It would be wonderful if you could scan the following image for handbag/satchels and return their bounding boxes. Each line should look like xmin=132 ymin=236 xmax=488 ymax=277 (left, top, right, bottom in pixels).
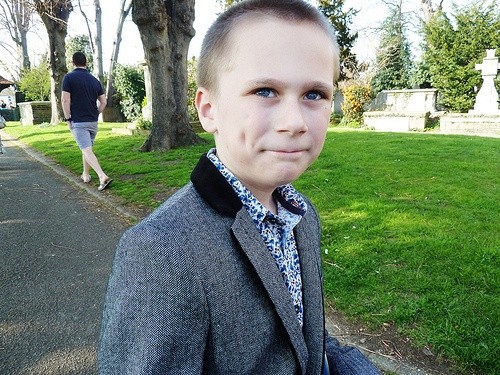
xmin=0 ymin=116 xmax=6 ymax=128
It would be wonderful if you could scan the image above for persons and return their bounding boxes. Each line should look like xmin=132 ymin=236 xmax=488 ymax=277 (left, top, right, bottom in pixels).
xmin=1 ymin=102 xmax=6 ymax=108
xmin=61 ymin=52 xmax=114 ymax=191
xmin=0 ymin=108 xmax=6 ymax=154
xmin=97 ymin=0 xmax=389 ymax=375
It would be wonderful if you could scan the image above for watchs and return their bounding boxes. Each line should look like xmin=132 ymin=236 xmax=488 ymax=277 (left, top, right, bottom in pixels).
xmin=64 ymin=118 xmax=71 ymax=122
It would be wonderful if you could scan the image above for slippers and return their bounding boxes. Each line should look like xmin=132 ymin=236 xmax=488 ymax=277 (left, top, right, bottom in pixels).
xmin=98 ymin=178 xmax=113 ymax=191
xmin=81 ymin=174 xmax=91 ymax=183
